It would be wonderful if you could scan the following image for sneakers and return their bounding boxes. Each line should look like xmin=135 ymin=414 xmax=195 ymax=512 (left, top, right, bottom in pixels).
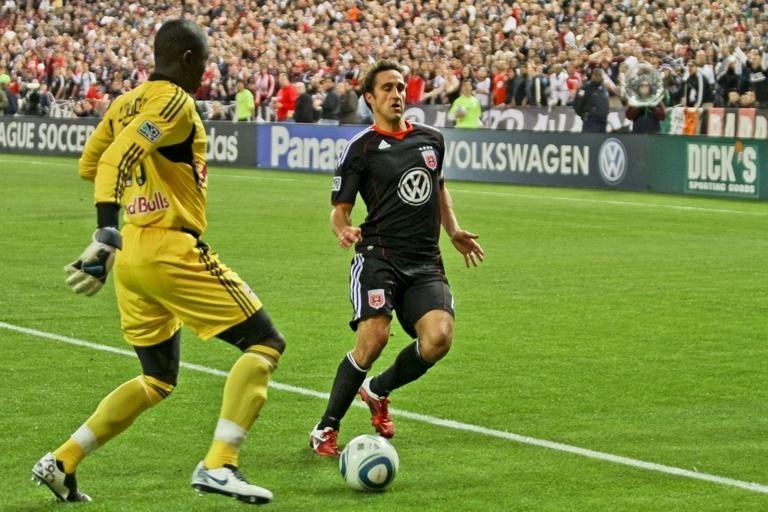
xmin=360 ymin=376 xmax=396 ymax=438
xmin=309 ymin=421 xmax=342 ymax=459
xmin=31 ymin=452 xmax=93 ymax=504
xmin=189 ymin=460 xmax=273 ymax=506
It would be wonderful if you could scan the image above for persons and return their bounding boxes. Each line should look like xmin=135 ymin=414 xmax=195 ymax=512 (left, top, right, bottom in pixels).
xmin=1 ymin=0 xmax=768 ymax=133
xmin=307 ymin=59 xmax=485 ymax=456
xmin=31 ymin=16 xmax=287 ymax=505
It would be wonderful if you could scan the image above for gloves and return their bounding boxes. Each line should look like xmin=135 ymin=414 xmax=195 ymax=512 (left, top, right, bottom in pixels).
xmin=63 ymin=227 xmax=124 ymax=298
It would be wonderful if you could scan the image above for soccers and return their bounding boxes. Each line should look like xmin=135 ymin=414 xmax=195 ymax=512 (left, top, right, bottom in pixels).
xmin=338 ymin=434 xmax=399 ymax=493
